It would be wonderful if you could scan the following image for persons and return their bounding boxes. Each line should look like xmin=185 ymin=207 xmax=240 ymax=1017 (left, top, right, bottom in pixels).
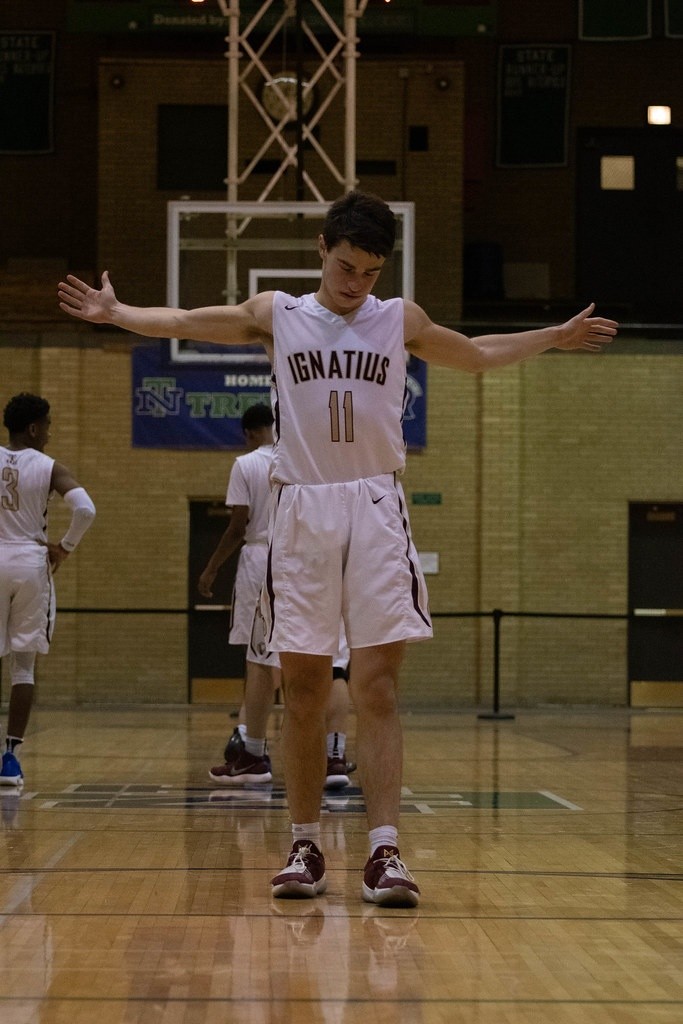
xmin=57 ymin=190 xmax=619 ymax=908
xmin=196 ymin=403 xmax=355 ymax=788
xmin=0 ymin=393 xmax=95 ymax=786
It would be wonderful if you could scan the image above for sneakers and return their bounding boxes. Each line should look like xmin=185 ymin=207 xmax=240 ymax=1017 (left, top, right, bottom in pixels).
xmin=361 ymin=845 xmax=421 ymax=905
xmin=324 ymin=755 xmax=349 ymax=788
xmin=361 ymin=901 xmax=420 ymax=962
xmin=270 ymin=840 xmax=327 ymax=897
xmin=209 ymin=789 xmax=270 ymax=826
xmin=320 ymin=795 xmax=349 ymax=814
xmin=207 ymin=748 xmax=273 ymax=783
xmin=1 ymin=786 xmax=23 ymax=820
xmin=345 ymin=761 xmax=357 ymax=774
xmin=269 ymin=905 xmax=325 ymax=948
xmin=0 ymin=753 xmax=24 ymax=786
xmin=225 ymin=727 xmax=271 ymax=770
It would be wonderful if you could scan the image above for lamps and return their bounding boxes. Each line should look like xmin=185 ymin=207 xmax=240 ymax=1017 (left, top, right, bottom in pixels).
xmin=257 ymin=0 xmax=319 ymax=125
